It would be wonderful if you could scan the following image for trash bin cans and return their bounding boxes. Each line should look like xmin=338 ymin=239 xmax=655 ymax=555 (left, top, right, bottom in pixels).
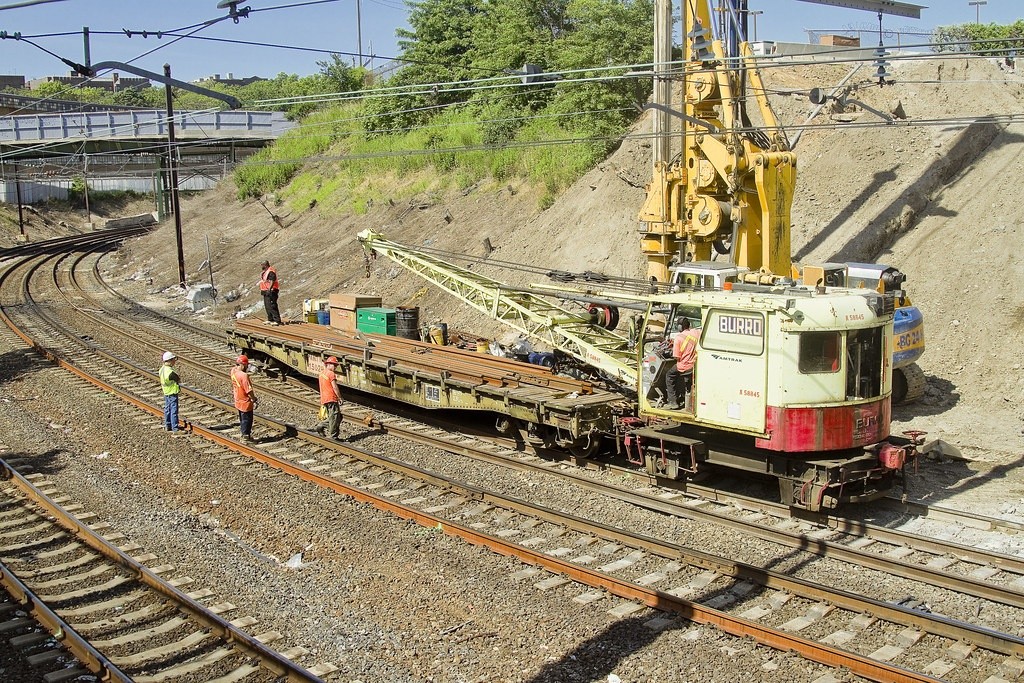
xmin=356 ymin=307 xmax=397 ymax=336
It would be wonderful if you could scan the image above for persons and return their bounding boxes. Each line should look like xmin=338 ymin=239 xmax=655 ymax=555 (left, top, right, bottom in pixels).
xmin=313 ymin=355 xmax=344 ymax=443
xmin=230 ymin=355 xmax=258 ymax=445
xmin=158 ymin=351 xmax=188 ymax=435
xmin=662 ymin=317 xmax=701 ymax=409
xmin=259 ymin=260 xmax=283 ymax=326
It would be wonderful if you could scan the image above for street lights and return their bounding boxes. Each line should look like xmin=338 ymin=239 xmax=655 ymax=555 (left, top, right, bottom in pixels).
xmin=967 ymin=0 xmax=988 ymax=24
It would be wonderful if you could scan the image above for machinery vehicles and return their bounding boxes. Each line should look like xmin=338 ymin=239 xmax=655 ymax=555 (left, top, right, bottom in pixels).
xmin=634 ymin=0 xmax=931 ymax=407
xmin=354 ymin=223 xmax=930 ymax=516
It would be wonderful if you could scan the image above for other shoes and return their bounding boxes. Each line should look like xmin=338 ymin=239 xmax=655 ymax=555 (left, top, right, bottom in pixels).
xmin=677 ymin=400 xmax=684 ymax=406
xmin=263 ymin=321 xmax=271 ymax=324
xmin=331 ymin=437 xmax=344 ymax=442
xmin=663 ymin=401 xmax=679 ymax=409
xmin=173 ymin=430 xmax=187 ymax=435
xmin=317 ymin=426 xmax=325 ymax=437
xmin=270 ymin=322 xmax=278 ymax=326
xmin=165 ymin=427 xmax=172 ymax=431
xmin=240 ymin=435 xmax=258 ymax=445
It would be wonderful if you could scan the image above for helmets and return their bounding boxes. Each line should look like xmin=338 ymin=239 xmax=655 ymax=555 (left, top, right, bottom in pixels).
xmin=324 ymin=356 xmax=340 ymax=365
xmin=163 ymin=351 xmax=176 ymax=362
xmin=236 ymin=355 xmax=249 ymax=365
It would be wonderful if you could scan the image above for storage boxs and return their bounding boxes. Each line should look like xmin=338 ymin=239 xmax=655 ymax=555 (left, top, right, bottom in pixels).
xmin=329 ymin=294 xmax=397 ymax=336
xmin=303 ymin=299 xmax=329 ymax=323
